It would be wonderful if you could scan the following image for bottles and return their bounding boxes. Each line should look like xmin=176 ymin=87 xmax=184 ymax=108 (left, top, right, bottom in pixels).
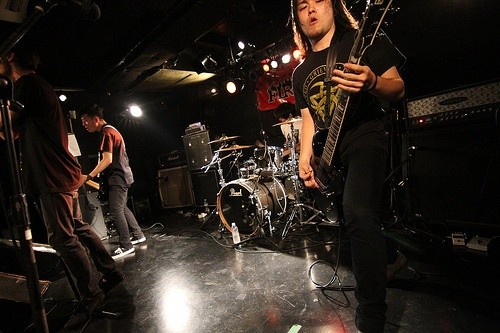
xmin=231 ymin=222 xmax=241 ymax=244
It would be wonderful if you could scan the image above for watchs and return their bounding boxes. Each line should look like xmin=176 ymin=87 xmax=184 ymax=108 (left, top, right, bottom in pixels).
xmin=87 ymin=174 xmax=93 ymax=180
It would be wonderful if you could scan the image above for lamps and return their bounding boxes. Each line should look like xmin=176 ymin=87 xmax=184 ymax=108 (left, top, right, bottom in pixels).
xmin=224 ymin=77 xmax=246 ymax=95
xmin=191 ymin=52 xmax=216 ymax=75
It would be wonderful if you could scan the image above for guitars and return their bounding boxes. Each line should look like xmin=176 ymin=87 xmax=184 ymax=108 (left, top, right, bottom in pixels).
xmin=305 ymin=1 xmax=393 ymax=223
xmin=85 ymin=178 xmax=102 ymax=194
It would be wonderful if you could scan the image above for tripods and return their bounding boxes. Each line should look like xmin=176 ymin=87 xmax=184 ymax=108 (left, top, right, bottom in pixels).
xmin=230 ymin=172 xmax=283 ymax=251
xmin=278 ymin=124 xmax=325 ymax=240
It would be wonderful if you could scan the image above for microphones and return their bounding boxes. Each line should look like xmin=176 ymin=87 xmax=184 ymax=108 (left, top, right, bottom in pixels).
xmin=73 ymin=1 xmax=101 ymax=21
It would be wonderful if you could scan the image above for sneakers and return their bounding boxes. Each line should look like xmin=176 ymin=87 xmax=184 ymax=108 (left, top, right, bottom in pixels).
xmin=96 ymin=269 xmax=126 ymax=293
xmin=64 ymin=291 xmax=106 ymax=329
xmin=378 ymin=250 xmax=408 ymax=283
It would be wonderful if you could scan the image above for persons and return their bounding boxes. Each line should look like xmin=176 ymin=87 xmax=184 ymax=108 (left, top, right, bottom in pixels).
xmin=74 ymin=106 xmax=146 ymax=260
xmin=274 ymin=101 xmax=301 ymax=140
xmin=286 ymin=0 xmax=403 ymax=333
xmin=7 ymin=42 xmax=126 ymax=309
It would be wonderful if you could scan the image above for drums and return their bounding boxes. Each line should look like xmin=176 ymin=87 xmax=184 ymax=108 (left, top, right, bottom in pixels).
xmin=215 ymin=171 xmax=286 ymax=239
xmin=282 ymin=173 xmax=304 ymax=201
xmin=256 ymin=146 xmax=286 ymax=178
xmin=239 ymin=174 xmax=258 ymax=179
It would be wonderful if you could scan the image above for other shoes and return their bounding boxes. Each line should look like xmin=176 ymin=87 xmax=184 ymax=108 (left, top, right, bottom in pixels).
xmin=129 ymin=234 xmax=147 ymax=245
xmin=110 ymin=246 xmax=135 ymax=261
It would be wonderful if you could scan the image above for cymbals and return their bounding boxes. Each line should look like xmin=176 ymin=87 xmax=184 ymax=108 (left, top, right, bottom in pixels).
xmin=214 ymin=144 xmax=253 ymax=154
xmin=207 ymin=135 xmax=240 ymax=145
xmin=272 ymin=119 xmax=302 ymax=126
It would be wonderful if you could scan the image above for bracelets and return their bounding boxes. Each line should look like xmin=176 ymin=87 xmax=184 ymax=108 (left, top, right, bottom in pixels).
xmin=369 ymin=76 xmax=377 ymax=92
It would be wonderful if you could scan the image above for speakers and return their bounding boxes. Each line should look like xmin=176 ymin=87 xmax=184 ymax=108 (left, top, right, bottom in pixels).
xmin=158 ymin=165 xmax=195 ymax=208
xmin=402 ymin=110 xmax=500 ymax=237
xmin=185 ymin=131 xmax=218 ymax=171
xmin=79 ymin=191 xmax=106 ymax=241
xmin=188 ymin=169 xmax=219 ymax=208
